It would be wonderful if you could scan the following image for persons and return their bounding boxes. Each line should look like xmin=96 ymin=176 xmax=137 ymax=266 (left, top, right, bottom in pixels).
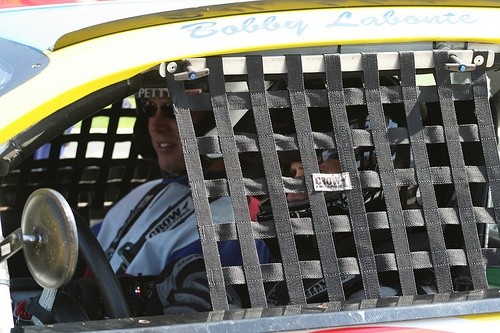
xmin=258 ymin=72 xmax=429 ymax=305
xmin=77 ymin=63 xmax=268 ymax=316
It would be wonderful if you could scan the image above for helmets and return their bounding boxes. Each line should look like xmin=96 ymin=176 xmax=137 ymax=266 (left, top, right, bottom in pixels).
xmin=232 ymin=75 xmax=425 ymax=256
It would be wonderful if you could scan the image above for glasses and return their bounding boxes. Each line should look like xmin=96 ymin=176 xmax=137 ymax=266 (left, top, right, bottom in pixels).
xmin=140 ymin=102 xmax=176 ymax=118
